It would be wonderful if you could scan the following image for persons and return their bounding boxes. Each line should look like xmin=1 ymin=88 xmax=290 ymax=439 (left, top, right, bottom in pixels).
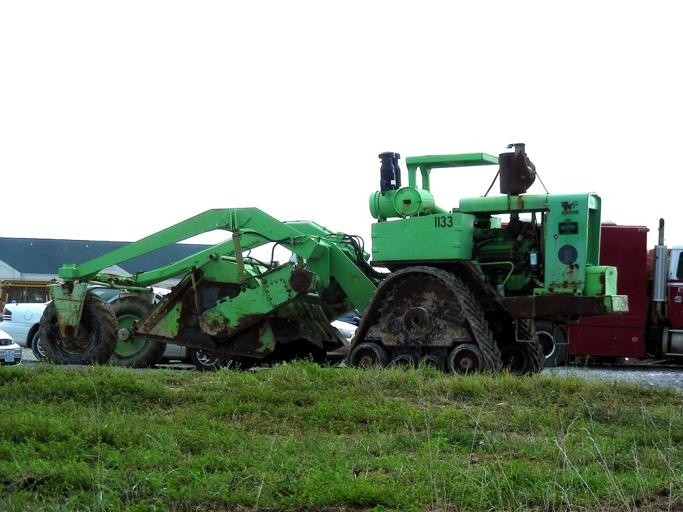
xmin=321 ymin=276 xmax=348 ymax=317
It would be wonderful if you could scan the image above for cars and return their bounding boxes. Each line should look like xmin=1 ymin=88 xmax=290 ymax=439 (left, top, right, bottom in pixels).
xmin=1 ymin=287 xmax=356 ymax=366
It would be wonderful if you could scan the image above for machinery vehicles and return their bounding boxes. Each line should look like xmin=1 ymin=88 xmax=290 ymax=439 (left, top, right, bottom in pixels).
xmin=41 ymin=144 xmax=628 ymax=375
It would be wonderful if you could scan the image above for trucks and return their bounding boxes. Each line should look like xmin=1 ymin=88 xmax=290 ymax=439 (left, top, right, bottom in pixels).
xmin=502 ymin=219 xmax=682 ymax=367
